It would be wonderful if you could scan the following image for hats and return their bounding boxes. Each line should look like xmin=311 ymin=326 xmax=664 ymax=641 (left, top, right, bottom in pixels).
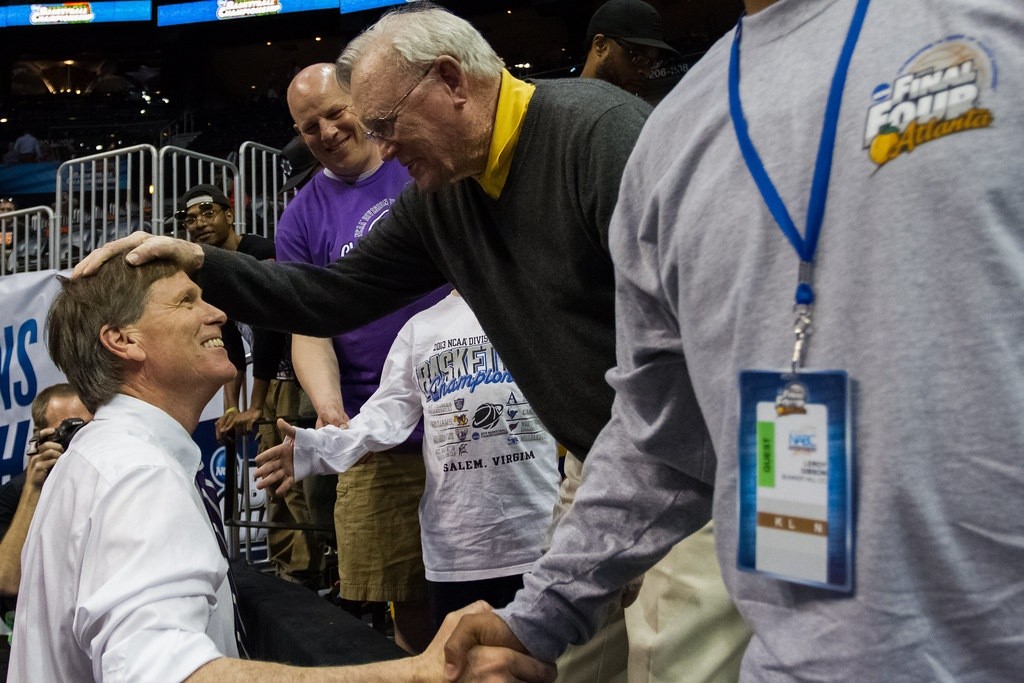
xmin=276 ymin=135 xmax=319 ymax=196
xmin=179 ymin=184 xmax=230 ymax=215
xmin=587 ymin=0 xmax=682 ymax=57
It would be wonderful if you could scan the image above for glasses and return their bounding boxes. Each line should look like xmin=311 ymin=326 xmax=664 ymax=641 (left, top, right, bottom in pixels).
xmin=605 ymin=37 xmax=652 ymax=68
xmin=175 ymin=203 xmax=226 ymax=228
xmin=360 ymin=53 xmax=450 ymax=145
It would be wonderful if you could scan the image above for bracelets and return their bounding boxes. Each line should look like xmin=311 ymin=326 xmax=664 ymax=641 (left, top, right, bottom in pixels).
xmin=224 ymin=407 xmax=239 ymax=415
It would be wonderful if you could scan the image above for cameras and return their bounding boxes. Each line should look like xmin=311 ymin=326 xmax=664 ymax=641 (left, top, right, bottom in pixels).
xmin=40 ymin=417 xmax=92 ymax=454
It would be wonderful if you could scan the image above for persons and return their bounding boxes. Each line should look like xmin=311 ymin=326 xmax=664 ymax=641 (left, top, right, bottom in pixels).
xmin=443 ymin=0 xmax=1024 ymax=683
xmin=0 ymin=0 xmax=657 ymax=683
xmin=4 ymin=246 xmax=560 ymax=683
xmin=13 ymin=127 xmax=42 ymax=162
